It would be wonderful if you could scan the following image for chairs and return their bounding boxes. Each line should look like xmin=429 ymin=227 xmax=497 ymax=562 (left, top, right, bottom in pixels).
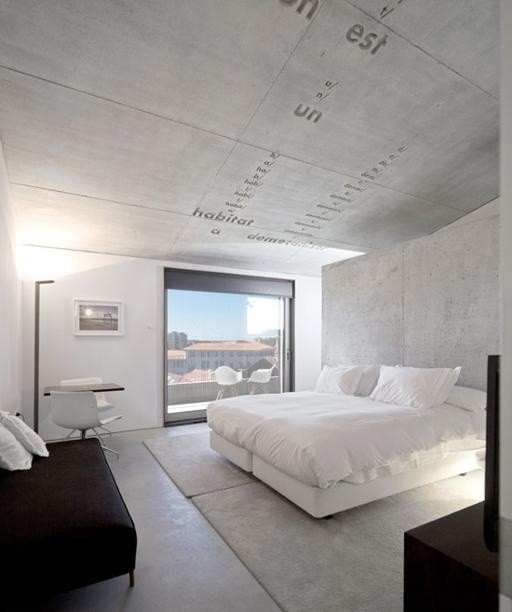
xmin=213 ymin=363 xmax=276 ymax=399
xmin=50 ymin=375 xmax=125 ymax=461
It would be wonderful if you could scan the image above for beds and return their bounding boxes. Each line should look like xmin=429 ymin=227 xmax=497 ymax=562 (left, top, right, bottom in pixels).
xmin=206 ymin=363 xmax=486 ymax=518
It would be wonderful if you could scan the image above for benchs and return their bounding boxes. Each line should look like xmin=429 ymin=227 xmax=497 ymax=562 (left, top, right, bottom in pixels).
xmin=1 ymin=437 xmax=137 ymax=609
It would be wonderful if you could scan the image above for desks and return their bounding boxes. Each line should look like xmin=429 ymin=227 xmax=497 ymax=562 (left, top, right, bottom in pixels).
xmin=43 ymin=381 xmax=125 ymax=396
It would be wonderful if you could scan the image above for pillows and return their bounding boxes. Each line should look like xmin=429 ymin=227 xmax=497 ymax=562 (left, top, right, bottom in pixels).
xmin=315 ymin=363 xmax=463 ymax=409
xmin=1 ymin=408 xmax=51 ymax=472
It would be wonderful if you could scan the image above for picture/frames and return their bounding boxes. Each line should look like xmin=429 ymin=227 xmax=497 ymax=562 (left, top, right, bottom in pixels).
xmin=72 ymin=297 xmax=126 ymax=336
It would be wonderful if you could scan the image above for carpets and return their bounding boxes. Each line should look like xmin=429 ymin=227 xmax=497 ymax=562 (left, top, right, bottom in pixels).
xmin=190 ymin=480 xmax=482 ymax=612
xmin=141 ymin=429 xmax=260 ymax=500
